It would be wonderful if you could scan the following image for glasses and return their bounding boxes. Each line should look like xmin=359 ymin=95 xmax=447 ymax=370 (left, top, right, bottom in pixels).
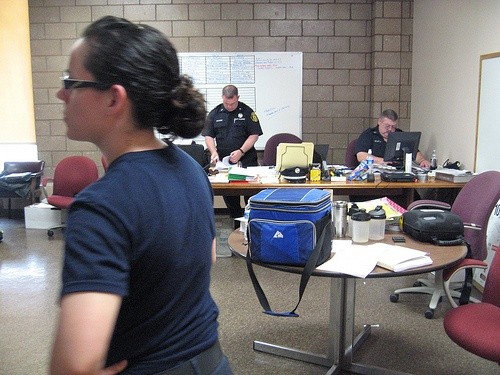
xmin=60 ymin=70 xmax=130 ymax=97
xmin=382 ymin=122 xmax=396 ymax=130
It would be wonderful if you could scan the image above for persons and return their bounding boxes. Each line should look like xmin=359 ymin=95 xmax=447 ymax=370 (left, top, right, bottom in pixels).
xmin=51 ymin=15 xmax=236 ymax=375
xmin=202 ymin=85 xmax=263 ymax=230
xmin=352 ymin=109 xmax=422 ymax=210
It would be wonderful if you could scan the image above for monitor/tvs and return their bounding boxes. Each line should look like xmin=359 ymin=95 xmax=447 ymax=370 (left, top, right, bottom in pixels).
xmin=385 ymin=131 xmax=421 ymax=173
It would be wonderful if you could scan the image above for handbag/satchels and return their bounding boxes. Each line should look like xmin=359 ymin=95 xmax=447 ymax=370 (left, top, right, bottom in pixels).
xmin=400 ymin=209 xmax=464 ymax=245
xmin=247 ymin=188 xmax=333 ymax=318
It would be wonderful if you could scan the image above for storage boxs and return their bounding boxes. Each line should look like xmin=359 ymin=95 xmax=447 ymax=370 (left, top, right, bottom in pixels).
xmin=24 ymin=203 xmax=63 ymax=229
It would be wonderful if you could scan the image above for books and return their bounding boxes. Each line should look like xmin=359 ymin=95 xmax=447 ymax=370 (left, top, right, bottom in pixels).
xmin=214 ymin=156 xmax=232 ymax=173
xmin=316 ymin=239 xmax=381 ymax=279
xmin=367 ymin=243 xmax=433 ymax=273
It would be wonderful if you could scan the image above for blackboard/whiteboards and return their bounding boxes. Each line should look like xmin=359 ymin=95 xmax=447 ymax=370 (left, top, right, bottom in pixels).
xmin=153 ymin=52 xmax=303 ymax=150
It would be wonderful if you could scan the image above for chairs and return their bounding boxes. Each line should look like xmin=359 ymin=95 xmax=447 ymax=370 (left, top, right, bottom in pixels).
xmin=442 ymin=241 xmax=500 ymax=366
xmin=263 ymin=133 xmax=361 ymax=168
xmin=0 ymin=159 xmax=45 ymax=217
xmin=42 ymin=156 xmax=98 ymax=237
xmin=389 ymin=170 xmax=500 ymax=319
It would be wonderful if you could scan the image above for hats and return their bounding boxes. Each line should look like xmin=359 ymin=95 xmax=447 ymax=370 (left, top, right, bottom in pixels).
xmin=281 ymin=166 xmax=308 ymax=183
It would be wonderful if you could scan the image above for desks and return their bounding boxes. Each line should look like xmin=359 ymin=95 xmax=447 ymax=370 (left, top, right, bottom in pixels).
xmin=227 ymin=221 xmax=469 ymax=375
xmin=207 ymin=167 xmax=472 ymax=210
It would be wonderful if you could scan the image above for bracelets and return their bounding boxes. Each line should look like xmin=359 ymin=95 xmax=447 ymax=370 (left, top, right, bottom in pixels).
xmin=239 ymin=148 xmax=245 ymax=156
xmin=419 ymin=159 xmax=427 ymax=165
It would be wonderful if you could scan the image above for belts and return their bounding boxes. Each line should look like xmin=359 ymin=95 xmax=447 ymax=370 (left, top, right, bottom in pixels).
xmin=154 ymin=341 xmax=224 ymax=375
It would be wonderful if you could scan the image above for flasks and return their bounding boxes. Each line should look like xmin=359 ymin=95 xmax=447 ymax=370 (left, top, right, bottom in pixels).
xmin=333 ymin=200 xmax=348 ymax=238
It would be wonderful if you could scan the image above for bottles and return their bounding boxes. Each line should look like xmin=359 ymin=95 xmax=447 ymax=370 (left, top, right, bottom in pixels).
xmin=368 ymin=205 xmax=386 ymax=241
xmin=430 ymin=150 xmax=437 ymax=170
xmin=365 ymin=149 xmax=374 ymax=165
xmin=348 ymin=204 xmax=359 ymax=238
xmin=351 ymin=208 xmax=372 ymax=243
